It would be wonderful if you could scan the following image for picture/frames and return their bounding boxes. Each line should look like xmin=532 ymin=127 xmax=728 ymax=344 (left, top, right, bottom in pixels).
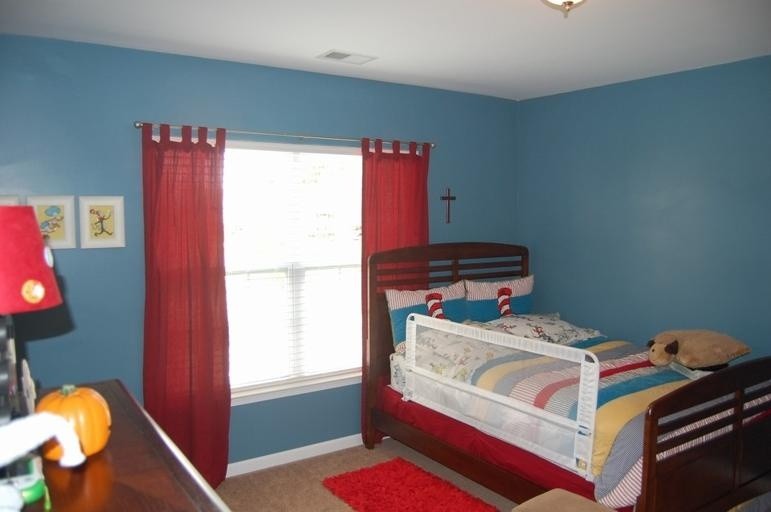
xmin=0 ymin=195 xmax=20 ymax=206
xmin=79 ymin=195 xmax=126 ymax=249
xmin=27 ymin=195 xmax=76 ymax=249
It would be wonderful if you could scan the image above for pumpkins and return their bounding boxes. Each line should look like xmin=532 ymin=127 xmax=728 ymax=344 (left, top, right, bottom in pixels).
xmin=40 ymin=450 xmax=117 ymax=511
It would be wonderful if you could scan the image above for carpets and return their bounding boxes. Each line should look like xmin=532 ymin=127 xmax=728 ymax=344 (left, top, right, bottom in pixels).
xmin=322 ymin=457 xmax=503 ymax=512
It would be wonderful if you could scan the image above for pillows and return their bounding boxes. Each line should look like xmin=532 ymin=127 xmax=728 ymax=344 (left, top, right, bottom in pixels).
xmin=395 ymin=319 xmax=522 ymax=377
xmin=481 ymin=312 xmax=604 ymax=348
xmin=653 ymin=327 xmax=751 ymax=368
xmin=463 ymin=274 xmax=535 ymax=325
xmin=384 ymin=280 xmax=466 ymax=346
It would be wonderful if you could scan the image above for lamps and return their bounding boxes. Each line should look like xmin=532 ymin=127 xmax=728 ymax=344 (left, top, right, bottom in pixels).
xmin=0 ymin=205 xmax=64 ymax=422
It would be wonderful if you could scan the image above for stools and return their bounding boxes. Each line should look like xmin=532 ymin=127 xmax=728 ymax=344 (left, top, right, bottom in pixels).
xmin=511 ymin=488 xmax=617 ymax=512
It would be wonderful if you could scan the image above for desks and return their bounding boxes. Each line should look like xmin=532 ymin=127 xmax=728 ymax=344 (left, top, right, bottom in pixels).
xmin=0 ymin=375 xmax=231 ymax=512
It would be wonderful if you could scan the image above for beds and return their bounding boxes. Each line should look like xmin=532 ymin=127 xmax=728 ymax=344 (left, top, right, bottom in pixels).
xmin=361 ymin=243 xmax=771 ymax=512
